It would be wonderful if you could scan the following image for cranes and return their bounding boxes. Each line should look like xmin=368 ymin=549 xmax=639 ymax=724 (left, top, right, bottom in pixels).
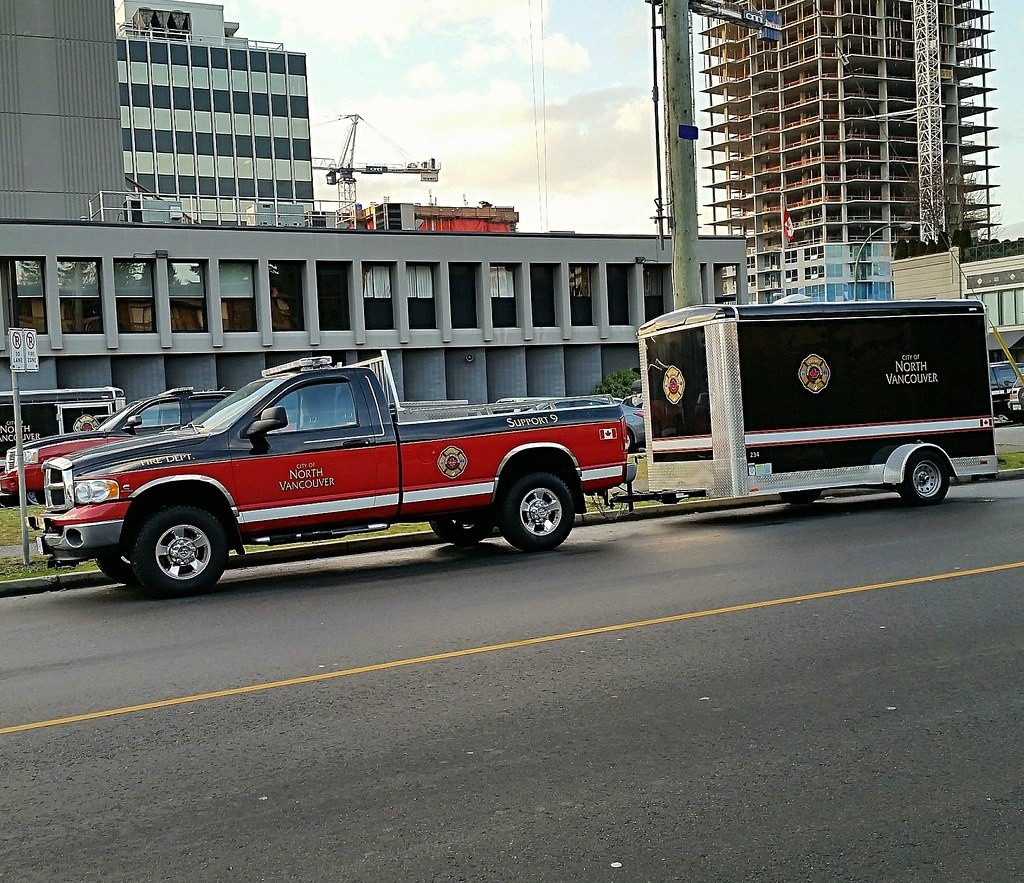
xmin=310 ymin=114 xmax=441 ymax=229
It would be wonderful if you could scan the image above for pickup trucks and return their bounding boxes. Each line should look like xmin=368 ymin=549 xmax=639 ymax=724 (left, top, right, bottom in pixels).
xmin=29 ymin=342 xmax=638 ymax=597
xmin=0 ymin=382 xmax=470 ymax=505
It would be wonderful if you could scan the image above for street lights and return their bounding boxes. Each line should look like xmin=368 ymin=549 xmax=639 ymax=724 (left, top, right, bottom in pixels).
xmin=854 ymin=221 xmax=912 ymax=303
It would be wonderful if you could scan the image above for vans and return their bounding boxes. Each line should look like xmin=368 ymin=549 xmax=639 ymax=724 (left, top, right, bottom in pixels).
xmin=491 ymin=396 xmax=624 ymax=413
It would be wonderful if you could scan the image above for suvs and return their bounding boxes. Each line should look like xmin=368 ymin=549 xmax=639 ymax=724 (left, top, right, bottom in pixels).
xmin=989 ymin=362 xmax=1024 ymax=416
xmin=1007 ymin=374 xmax=1023 ymax=425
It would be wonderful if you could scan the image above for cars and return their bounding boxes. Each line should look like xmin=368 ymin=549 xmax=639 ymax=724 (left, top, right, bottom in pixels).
xmin=520 ymin=397 xmax=644 ymax=453
